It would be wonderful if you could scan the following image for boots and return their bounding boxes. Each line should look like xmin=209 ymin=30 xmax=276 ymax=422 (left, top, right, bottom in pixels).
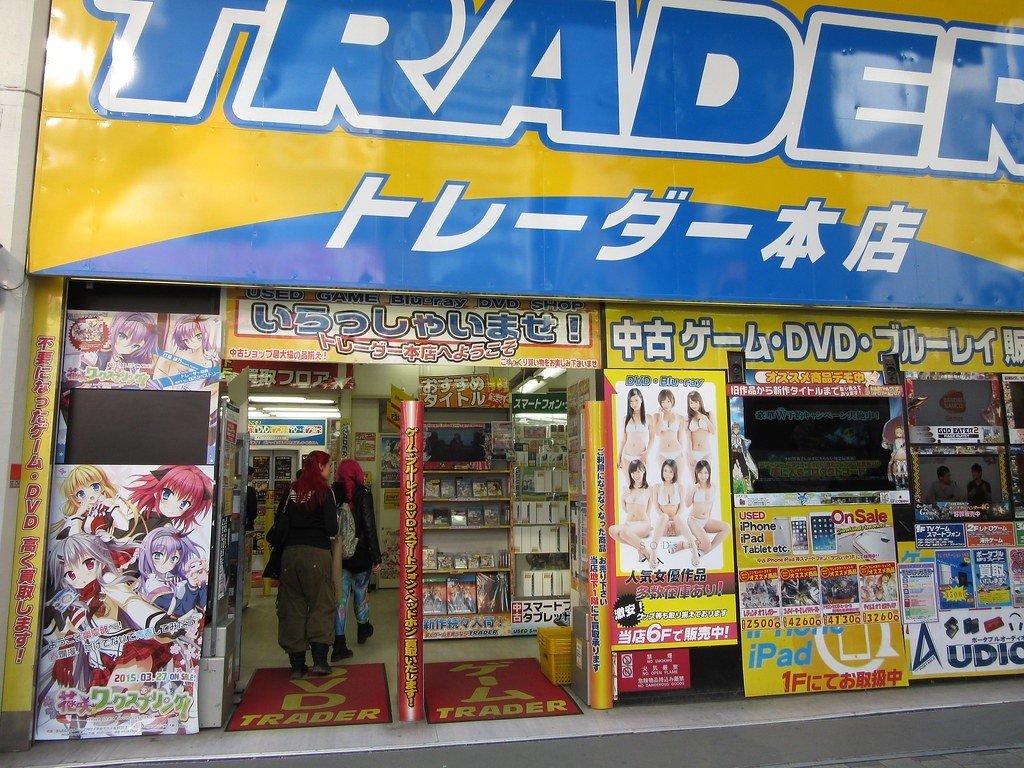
xmin=288 ymin=651 xmax=308 ymax=677
xmin=357 ymin=620 xmax=373 ymax=643
xmin=311 ymin=643 xmax=332 ymax=673
xmin=331 ymin=634 xmax=353 ymax=661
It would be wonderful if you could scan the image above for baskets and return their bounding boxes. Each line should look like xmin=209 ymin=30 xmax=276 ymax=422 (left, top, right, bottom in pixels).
xmin=536 ymin=626 xmax=573 ymax=685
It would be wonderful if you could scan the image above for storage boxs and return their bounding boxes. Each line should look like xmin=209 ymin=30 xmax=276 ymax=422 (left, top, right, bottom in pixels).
xmin=537 ymin=625 xmax=573 ymax=684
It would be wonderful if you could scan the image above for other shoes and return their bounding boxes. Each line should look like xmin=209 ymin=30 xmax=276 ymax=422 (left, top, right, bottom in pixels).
xmin=668 ymin=542 xmax=684 ymax=554
xmin=691 ymin=544 xmax=701 ymax=567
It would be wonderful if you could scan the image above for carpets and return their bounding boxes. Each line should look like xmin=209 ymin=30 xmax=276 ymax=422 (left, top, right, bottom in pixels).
xmin=425 ymin=657 xmax=584 ymax=726
xmin=223 ymin=662 xmax=393 ymax=734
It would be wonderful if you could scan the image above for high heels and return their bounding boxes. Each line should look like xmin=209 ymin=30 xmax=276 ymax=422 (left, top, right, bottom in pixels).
xmin=639 ymin=551 xmax=646 ymax=562
xmin=648 ymin=557 xmax=664 ymax=569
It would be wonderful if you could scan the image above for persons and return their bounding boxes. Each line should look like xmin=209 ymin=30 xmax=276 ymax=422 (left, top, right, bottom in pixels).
xmin=926 ymin=465 xmax=965 ymax=502
xmin=330 ymin=458 xmax=382 ymax=661
xmin=649 ymin=458 xmax=701 ymax=569
xmin=617 ymin=387 xmax=654 ymax=484
xmin=608 ymin=458 xmax=663 ymax=570
xmin=653 ymin=389 xmax=685 ymax=482
xmin=668 ymin=459 xmax=731 ymax=566
xmin=685 ymin=390 xmax=717 ymax=484
xmin=245 ymin=466 xmax=259 ymax=530
xmin=274 ymin=450 xmax=339 ymax=678
xmin=962 ymin=460 xmax=992 ymax=503
xmin=262 ymin=469 xmax=302 ymax=581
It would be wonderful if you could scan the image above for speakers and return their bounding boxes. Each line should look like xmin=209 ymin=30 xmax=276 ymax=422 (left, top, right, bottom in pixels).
xmin=726 ymin=352 xmax=745 ymax=384
xmin=881 ymin=356 xmax=901 ymax=385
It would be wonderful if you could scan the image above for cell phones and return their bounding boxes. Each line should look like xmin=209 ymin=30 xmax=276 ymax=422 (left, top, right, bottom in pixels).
xmin=791 ymin=517 xmax=809 ymax=556
xmin=772 ymin=517 xmax=790 ymax=556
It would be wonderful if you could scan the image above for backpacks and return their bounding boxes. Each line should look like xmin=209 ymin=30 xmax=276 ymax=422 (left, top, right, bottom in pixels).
xmin=336 ymin=502 xmax=358 ymax=558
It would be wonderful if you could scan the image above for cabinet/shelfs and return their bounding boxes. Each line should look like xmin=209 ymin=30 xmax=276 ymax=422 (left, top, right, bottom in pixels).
xmin=511 ymin=461 xmax=568 ymax=600
xmin=422 ymin=470 xmax=512 ymax=575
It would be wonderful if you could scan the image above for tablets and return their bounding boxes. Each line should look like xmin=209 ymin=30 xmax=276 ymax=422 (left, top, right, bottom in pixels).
xmin=809 ymin=512 xmax=837 ymax=555
